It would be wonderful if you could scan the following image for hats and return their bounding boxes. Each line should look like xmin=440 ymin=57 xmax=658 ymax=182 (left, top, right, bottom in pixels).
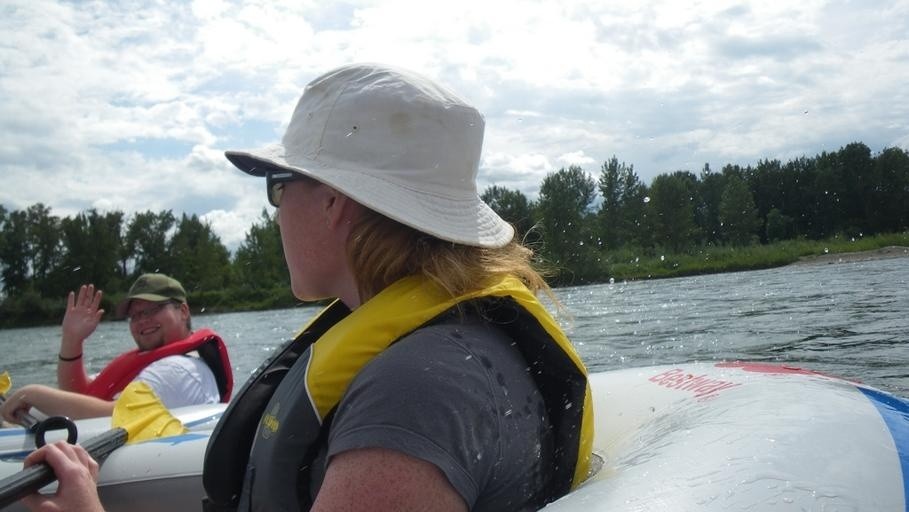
xmin=224 ymin=61 xmax=516 ymax=249
xmin=116 ymin=273 xmax=187 ymax=320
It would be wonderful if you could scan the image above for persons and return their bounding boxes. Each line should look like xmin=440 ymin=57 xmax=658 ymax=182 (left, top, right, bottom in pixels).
xmin=23 ymin=60 xmax=603 ymax=512
xmin=0 ymin=274 xmax=233 ymax=423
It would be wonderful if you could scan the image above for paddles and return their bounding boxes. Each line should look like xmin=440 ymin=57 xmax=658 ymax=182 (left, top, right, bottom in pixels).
xmin=1 ymin=380 xmax=188 ymax=509
xmin=0 ymin=371 xmax=37 ymax=430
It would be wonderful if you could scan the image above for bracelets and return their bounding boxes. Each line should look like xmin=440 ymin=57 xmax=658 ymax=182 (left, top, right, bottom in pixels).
xmin=58 ymin=355 xmax=85 ymax=362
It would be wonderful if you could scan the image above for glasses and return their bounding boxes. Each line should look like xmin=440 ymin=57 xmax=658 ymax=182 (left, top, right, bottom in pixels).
xmin=124 ymin=301 xmax=171 ymax=323
xmin=266 ymin=168 xmax=300 ymax=207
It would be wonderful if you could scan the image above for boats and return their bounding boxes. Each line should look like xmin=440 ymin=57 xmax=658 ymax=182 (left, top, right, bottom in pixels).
xmin=0 ymin=362 xmax=909 ymax=512
